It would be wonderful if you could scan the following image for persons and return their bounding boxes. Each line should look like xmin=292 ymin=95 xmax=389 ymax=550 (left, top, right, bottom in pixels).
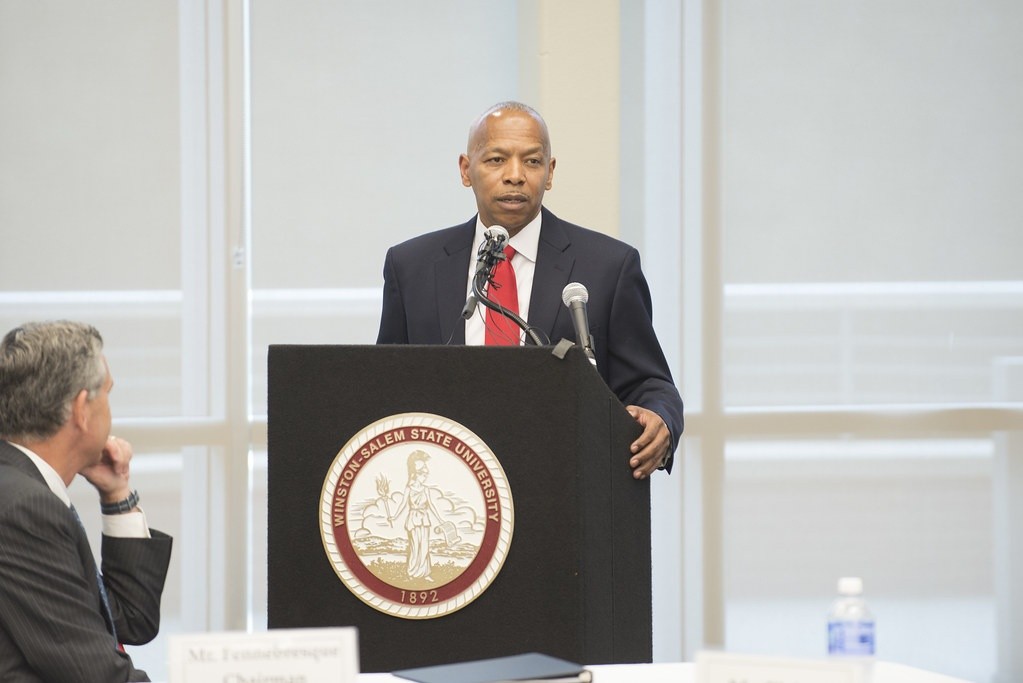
xmin=0 ymin=318 xmax=173 ymax=683
xmin=376 ymin=101 xmax=684 ymax=480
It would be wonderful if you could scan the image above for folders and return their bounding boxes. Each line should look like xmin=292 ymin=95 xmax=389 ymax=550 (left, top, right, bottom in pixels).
xmin=392 ymin=650 xmax=596 ymax=683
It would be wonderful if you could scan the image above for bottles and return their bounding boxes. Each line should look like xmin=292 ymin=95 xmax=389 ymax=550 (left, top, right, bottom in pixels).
xmin=826 ymin=575 xmax=877 ymax=682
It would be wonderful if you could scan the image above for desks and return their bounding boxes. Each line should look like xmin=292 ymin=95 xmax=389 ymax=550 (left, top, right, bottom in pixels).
xmin=357 ymin=655 xmax=973 ymax=683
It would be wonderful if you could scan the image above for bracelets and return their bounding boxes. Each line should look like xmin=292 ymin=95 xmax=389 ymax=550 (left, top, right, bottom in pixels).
xmin=100 ymin=489 xmax=140 ymax=514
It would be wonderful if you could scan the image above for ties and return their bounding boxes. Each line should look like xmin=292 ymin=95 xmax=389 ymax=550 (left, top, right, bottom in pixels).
xmin=484 ymin=244 xmax=520 ymax=346
xmin=69 ymin=502 xmax=119 ymax=653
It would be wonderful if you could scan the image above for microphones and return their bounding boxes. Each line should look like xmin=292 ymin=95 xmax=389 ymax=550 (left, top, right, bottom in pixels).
xmin=561 ymin=282 xmax=597 ymax=371
xmin=460 ymin=225 xmax=509 ymax=320
